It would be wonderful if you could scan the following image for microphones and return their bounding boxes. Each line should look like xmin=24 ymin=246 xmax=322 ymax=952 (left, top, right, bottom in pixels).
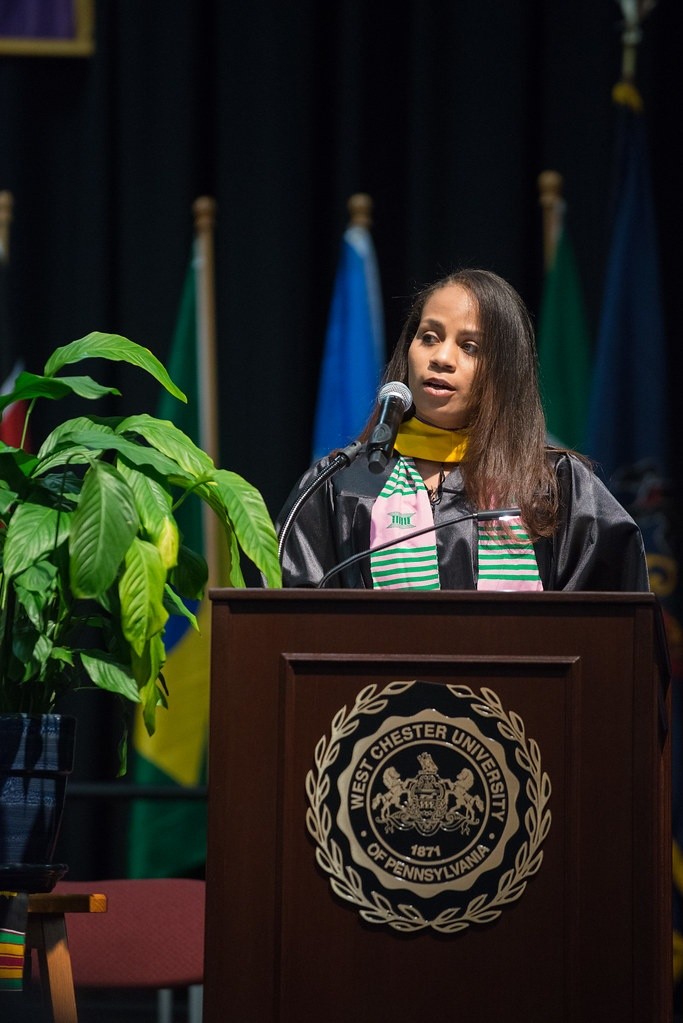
xmin=365 ymin=381 xmax=414 ymax=475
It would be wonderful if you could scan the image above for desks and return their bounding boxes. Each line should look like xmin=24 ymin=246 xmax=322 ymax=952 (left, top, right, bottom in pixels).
xmin=0 ymin=894 xmax=109 ymax=1023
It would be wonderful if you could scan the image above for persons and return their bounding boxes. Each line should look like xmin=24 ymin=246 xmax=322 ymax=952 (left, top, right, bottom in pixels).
xmin=261 ymin=269 xmax=650 ymax=593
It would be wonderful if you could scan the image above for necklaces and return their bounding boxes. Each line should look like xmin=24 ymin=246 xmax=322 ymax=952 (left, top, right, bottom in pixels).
xmin=428 ymin=462 xmax=446 ymax=505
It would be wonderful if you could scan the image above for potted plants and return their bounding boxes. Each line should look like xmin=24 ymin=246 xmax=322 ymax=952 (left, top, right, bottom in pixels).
xmin=0 ymin=329 xmax=283 ymax=893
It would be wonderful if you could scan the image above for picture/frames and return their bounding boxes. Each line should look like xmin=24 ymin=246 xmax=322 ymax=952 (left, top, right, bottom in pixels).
xmin=0 ymin=0 xmax=96 ymax=58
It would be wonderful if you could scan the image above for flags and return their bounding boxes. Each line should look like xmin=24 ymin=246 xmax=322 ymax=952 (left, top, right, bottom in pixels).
xmin=312 ymin=193 xmax=382 ymax=464
xmin=127 ymin=193 xmax=231 ymax=879
xmin=535 ymin=72 xmax=683 ymax=989
